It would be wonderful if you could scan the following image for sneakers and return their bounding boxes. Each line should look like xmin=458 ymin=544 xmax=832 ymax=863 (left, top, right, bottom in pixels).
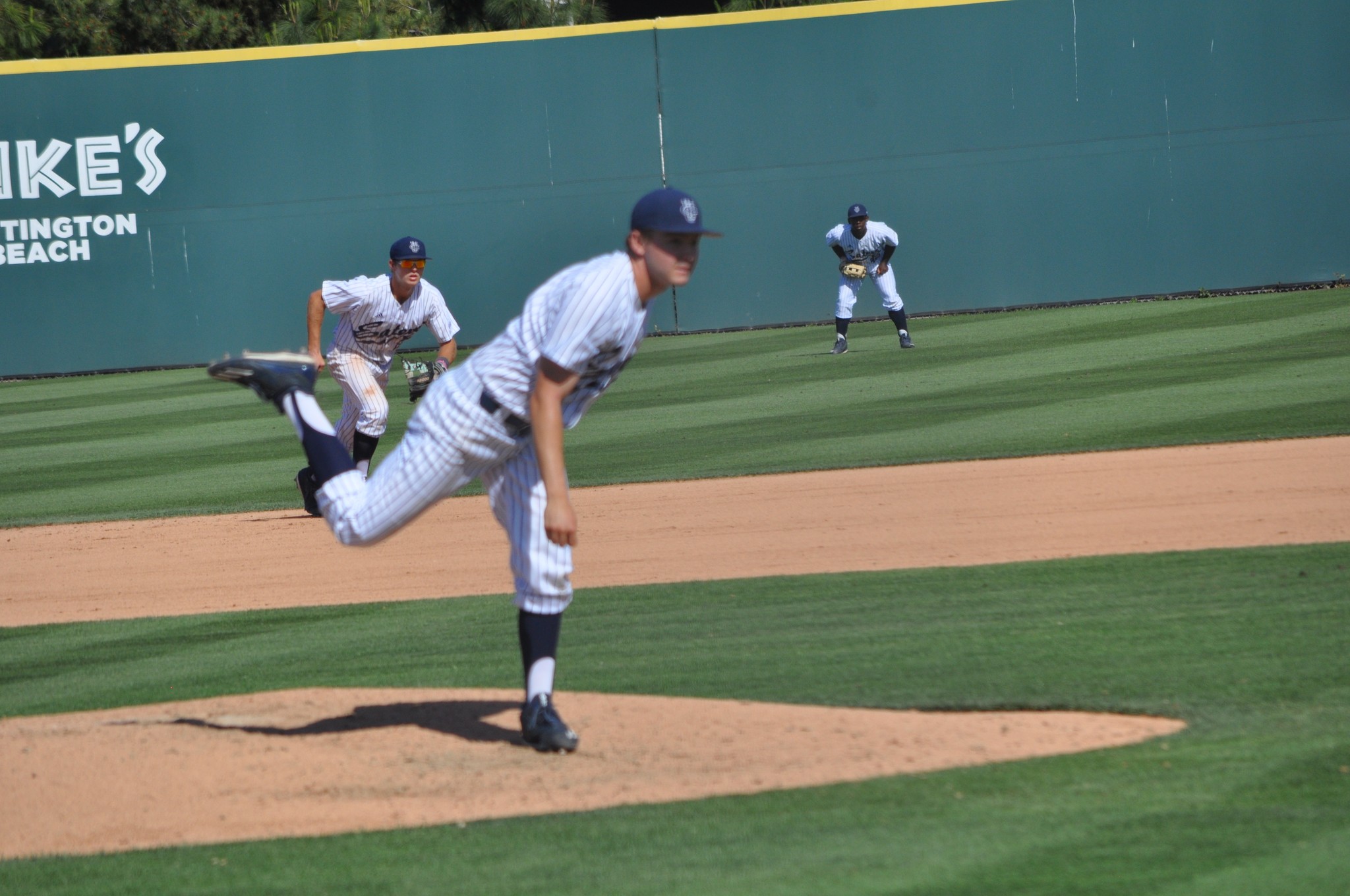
xmin=830 ymin=337 xmax=848 ymax=354
xmin=899 ymin=333 xmax=915 ymax=348
xmin=208 ymin=350 xmax=317 ymax=401
xmin=294 ymin=466 xmax=321 ymax=516
xmin=519 ymin=694 xmax=577 ymax=752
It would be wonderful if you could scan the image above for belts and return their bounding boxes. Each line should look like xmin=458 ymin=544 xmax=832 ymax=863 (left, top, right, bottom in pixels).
xmin=479 ymin=391 xmax=531 ymax=439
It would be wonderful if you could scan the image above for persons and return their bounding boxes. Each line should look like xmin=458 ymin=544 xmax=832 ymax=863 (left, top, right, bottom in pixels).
xmin=207 ymin=191 xmax=725 ymax=752
xmin=294 ymin=236 xmax=460 ymax=517
xmin=825 ymin=204 xmax=916 ymax=355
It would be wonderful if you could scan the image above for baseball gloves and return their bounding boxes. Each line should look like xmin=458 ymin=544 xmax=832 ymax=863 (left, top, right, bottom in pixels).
xmin=404 ymin=359 xmax=445 ymax=402
xmin=843 ymin=261 xmax=867 ymax=281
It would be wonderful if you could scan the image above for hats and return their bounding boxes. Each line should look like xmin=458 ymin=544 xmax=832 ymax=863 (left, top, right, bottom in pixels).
xmin=390 ymin=236 xmax=432 ymax=260
xmin=847 ymin=204 xmax=868 ymax=219
xmin=632 ymin=187 xmax=719 ymax=238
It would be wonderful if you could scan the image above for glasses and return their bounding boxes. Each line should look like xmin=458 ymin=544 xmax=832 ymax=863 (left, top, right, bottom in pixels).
xmin=395 ymin=260 xmax=425 ymax=269
